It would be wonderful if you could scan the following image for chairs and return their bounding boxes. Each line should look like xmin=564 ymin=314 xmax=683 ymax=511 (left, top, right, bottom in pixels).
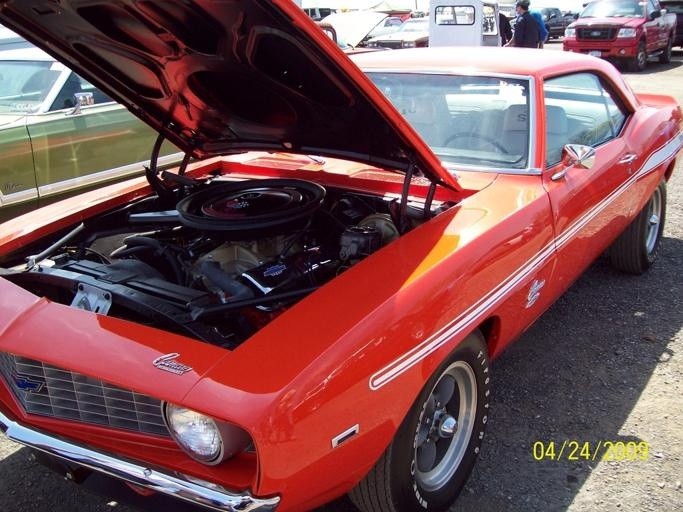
xmin=415 ymin=96 xmax=570 ymax=165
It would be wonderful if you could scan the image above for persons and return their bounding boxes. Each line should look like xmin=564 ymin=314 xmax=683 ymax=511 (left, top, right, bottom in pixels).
xmin=517 ymin=0 xmax=549 ymax=50
xmin=503 ymin=0 xmax=540 ymax=48
xmin=482 ymin=7 xmax=512 ymax=47
